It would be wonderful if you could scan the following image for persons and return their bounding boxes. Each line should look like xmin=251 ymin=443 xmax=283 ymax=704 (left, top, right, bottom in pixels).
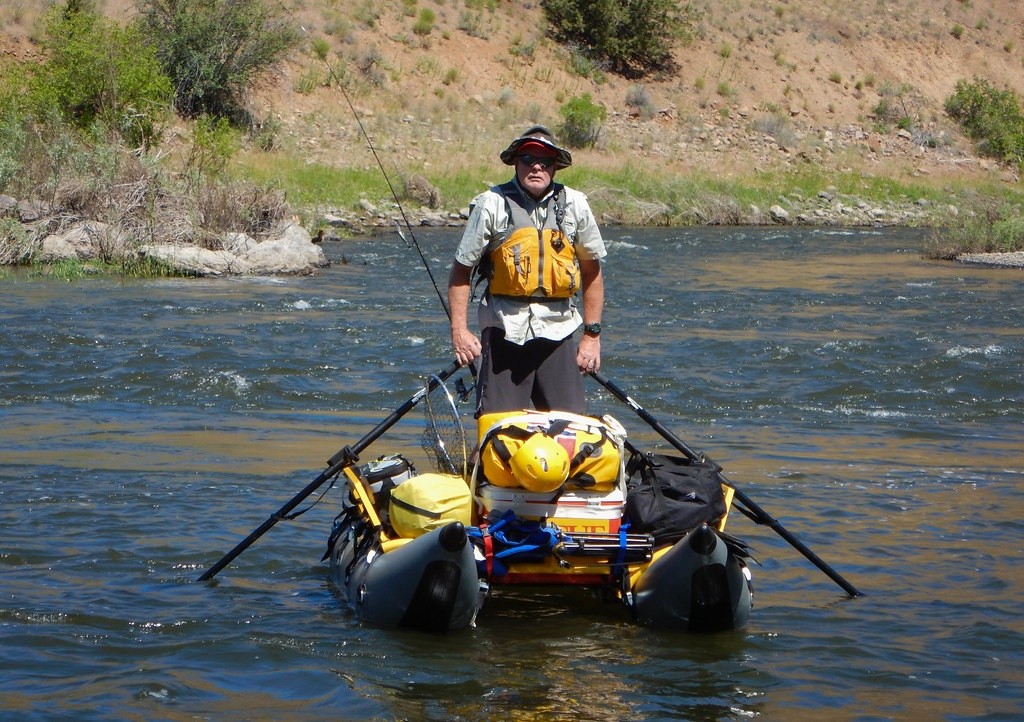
xmin=449 ymin=124 xmax=607 ymax=418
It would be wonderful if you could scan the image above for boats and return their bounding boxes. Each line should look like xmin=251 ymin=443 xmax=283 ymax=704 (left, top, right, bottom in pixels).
xmin=331 ymin=406 xmax=759 ymax=638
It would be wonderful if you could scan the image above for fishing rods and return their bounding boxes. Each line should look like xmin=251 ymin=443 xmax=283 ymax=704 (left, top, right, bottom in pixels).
xmin=274 ymin=0 xmax=483 ymax=395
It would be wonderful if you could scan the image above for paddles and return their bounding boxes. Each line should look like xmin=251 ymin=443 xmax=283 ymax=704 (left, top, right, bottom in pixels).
xmin=196 ymin=338 xmax=483 ymax=587
xmin=591 ymin=365 xmax=864 ymax=597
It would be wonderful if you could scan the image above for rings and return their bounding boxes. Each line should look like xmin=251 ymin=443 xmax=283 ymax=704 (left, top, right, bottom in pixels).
xmin=587 ymin=363 xmax=594 ymax=369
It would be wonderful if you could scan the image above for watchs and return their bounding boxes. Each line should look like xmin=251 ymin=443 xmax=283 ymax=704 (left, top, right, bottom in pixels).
xmin=585 ymin=322 xmax=601 ymax=334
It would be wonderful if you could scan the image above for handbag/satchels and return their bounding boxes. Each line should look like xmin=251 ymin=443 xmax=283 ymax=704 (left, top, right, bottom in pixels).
xmin=625 ymin=440 xmax=727 ymax=541
xmin=478 ymin=410 xmax=624 ymax=494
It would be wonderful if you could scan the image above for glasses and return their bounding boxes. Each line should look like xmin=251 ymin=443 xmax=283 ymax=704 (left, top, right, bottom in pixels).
xmin=514 ymin=152 xmax=556 ymax=170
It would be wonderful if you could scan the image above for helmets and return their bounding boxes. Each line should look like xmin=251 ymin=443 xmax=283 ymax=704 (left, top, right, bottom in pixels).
xmin=506 ymin=431 xmax=570 ymax=492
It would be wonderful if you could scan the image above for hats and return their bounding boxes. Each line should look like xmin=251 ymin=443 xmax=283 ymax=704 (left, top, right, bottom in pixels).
xmin=500 ymin=125 xmax=572 ymax=171
